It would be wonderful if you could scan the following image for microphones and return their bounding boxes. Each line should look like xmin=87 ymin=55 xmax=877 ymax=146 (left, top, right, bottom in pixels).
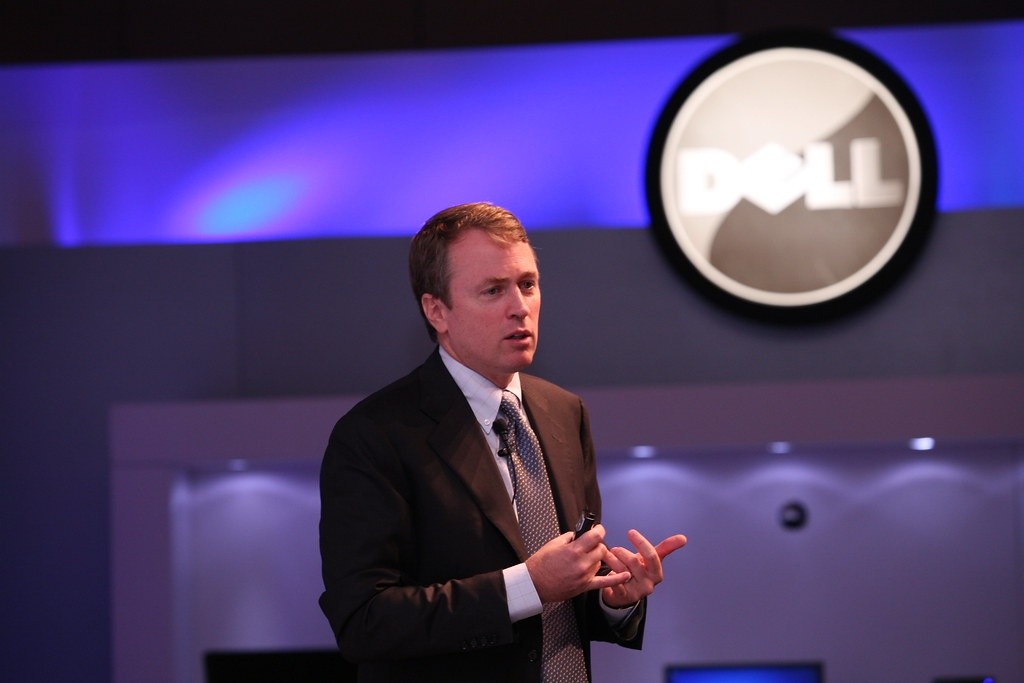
xmin=491 ymin=417 xmax=509 ymax=442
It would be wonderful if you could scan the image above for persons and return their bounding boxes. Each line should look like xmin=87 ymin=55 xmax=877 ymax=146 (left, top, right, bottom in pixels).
xmin=318 ymin=201 xmax=686 ymax=683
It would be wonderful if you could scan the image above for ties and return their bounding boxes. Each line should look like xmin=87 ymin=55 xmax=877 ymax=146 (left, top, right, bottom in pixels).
xmin=498 ymin=390 xmax=589 ymax=683
